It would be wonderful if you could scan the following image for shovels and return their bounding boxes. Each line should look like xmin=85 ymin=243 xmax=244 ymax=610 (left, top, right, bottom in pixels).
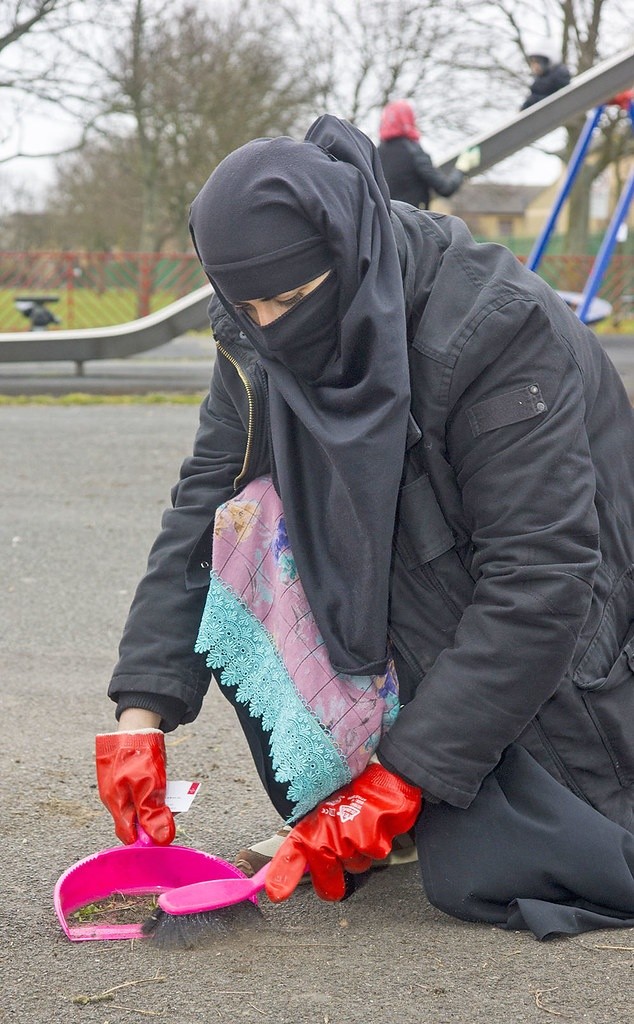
xmin=53 ymin=816 xmax=258 ymax=941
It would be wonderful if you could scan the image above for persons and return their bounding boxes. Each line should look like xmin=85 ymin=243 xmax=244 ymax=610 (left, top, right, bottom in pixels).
xmin=94 ymin=114 xmax=634 ymax=944
xmin=378 ymin=102 xmax=481 ymax=209
xmin=519 ymin=55 xmax=573 ymax=110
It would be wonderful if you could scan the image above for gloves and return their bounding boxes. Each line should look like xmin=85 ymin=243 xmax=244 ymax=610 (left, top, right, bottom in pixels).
xmin=95 ymin=729 xmax=174 ymax=848
xmin=263 ymin=762 xmax=424 ymax=904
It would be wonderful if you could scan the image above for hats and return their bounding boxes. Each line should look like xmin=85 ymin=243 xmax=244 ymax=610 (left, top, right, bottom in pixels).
xmin=377 ymin=101 xmax=421 ymax=140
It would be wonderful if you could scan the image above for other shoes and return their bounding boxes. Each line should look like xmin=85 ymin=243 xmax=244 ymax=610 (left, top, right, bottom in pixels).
xmin=233 ymin=822 xmax=418 ymax=887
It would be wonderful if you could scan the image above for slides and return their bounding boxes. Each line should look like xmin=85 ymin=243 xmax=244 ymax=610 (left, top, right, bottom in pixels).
xmin=0 ymin=46 xmax=634 ymax=362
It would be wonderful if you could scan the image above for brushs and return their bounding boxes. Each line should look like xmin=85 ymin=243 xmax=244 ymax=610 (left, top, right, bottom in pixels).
xmin=139 ymin=859 xmax=311 ymax=951
